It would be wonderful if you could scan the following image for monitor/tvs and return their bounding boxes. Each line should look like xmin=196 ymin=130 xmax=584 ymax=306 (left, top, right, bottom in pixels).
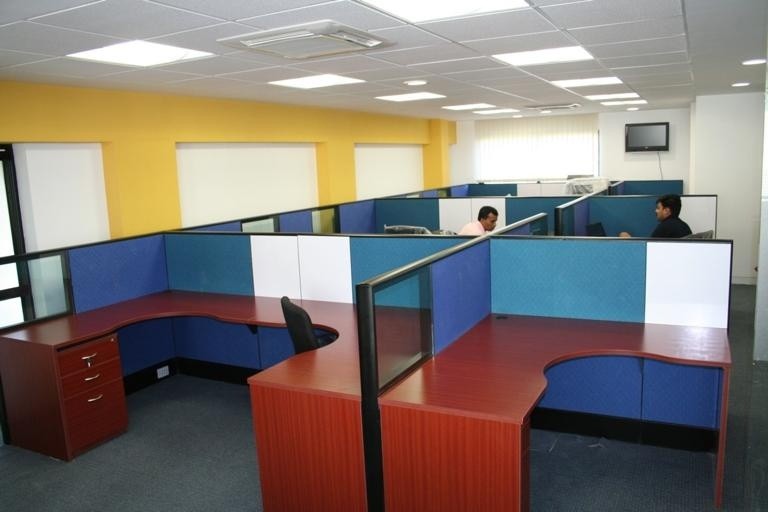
xmin=625 ymin=121 xmax=670 ymax=153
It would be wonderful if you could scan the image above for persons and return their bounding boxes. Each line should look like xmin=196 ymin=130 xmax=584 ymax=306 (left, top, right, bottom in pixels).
xmin=457 ymin=206 xmax=499 ymax=236
xmin=618 ymin=194 xmax=693 ymax=238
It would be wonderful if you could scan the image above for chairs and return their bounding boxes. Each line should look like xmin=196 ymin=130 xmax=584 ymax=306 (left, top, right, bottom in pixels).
xmin=281 ymin=297 xmax=319 ymax=355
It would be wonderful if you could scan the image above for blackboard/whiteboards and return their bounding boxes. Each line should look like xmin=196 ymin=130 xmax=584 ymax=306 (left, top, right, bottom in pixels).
xmin=472 ymin=112 xmax=600 ymax=184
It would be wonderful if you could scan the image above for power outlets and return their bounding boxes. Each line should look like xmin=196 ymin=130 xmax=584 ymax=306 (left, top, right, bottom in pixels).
xmin=157 ymin=366 xmax=169 ymax=379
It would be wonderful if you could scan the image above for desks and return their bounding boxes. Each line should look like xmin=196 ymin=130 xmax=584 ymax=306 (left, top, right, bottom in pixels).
xmin=0 ymin=289 xmax=431 ymax=512
xmin=377 ymin=312 xmax=732 ymax=512
